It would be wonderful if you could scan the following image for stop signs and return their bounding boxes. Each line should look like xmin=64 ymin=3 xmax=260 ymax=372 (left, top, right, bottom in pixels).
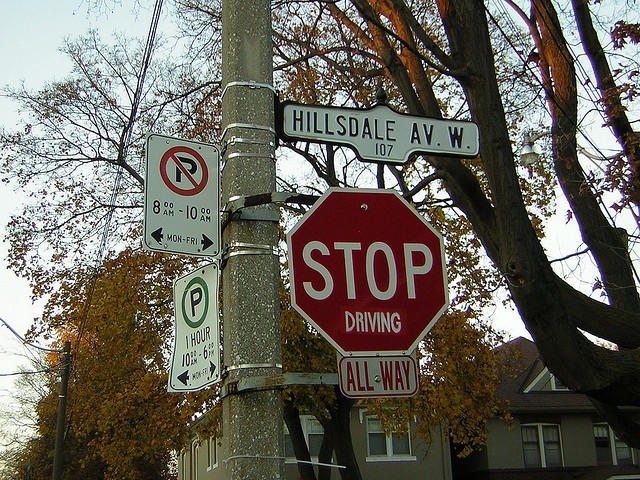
xmin=168 ymin=264 xmax=217 ymax=391
xmin=288 ymin=189 xmax=448 ymax=358
xmin=145 ymin=134 xmax=220 ymax=255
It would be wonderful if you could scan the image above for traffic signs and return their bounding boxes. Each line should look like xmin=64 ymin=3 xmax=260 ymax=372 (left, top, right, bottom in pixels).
xmin=282 ymin=95 xmax=479 ymax=165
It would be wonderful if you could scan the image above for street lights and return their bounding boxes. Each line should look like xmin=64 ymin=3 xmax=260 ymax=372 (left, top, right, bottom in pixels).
xmin=522 ymin=129 xmax=640 ymax=182
